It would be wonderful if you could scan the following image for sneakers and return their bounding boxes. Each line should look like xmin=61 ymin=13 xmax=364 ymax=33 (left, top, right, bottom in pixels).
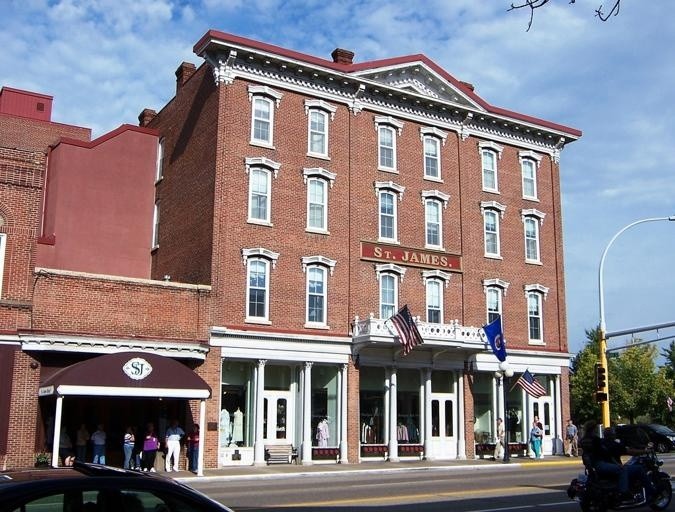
xmin=131 ymin=466 xmax=156 ymax=472
xmin=167 ymin=468 xmax=171 ymax=472
xmin=173 ymin=467 xmax=179 ymax=472
xmin=192 ymin=471 xmax=197 ymax=474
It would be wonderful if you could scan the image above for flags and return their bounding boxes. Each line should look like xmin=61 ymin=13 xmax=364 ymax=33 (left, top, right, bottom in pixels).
xmin=391 ymin=304 xmax=426 ymax=360
xmin=483 ymin=316 xmax=508 ymax=362
xmin=518 ymin=369 xmax=546 ymax=399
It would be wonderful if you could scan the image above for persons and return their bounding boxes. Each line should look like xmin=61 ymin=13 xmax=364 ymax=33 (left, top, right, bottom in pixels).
xmin=186 ymin=424 xmax=200 ymax=474
xmin=601 ymin=426 xmax=655 ymax=490
xmin=164 ymin=419 xmax=185 ymax=473
xmin=507 ymin=410 xmax=519 ymax=442
xmin=91 ymin=422 xmax=107 ymax=465
xmin=315 ymin=416 xmax=330 ymax=447
xmin=534 ymin=415 xmax=544 ymax=459
xmin=131 ymin=423 xmax=142 ymax=471
xmin=529 ymin=422 xmax=541 ymax=460
xmin=123 ymin=425 xmax=135 ymax=469
xmin=143 ymin=422 xmax=161 ymax=472
xmin=566 ymin=420 xmax=579 ymax=457
xmin=221 ymin=409 xmax=231 ymax=444
xmin=76 ymin=423 xmax=91 ymax=463
xmin=233 ymin=410 xmax=244 ymax=442
xmin=492 ymin=418 xmax=505 ymax=460
xmin=580 ymin=421 xmax=633 ymax=501
xmin=59 ymin=423 xmax=72 ymax=464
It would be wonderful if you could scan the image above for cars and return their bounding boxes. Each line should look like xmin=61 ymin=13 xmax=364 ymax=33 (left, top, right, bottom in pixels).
xmin=0 ymin=462 xmax=236 ymax=511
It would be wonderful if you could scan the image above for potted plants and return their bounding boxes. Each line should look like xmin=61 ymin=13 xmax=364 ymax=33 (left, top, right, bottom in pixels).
xmin=34 ymin=451 xmax=50 ymax=467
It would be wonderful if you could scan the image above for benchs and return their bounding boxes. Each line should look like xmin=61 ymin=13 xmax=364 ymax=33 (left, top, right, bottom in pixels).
xmin=264 ymin=444 xmax=298 ymax=464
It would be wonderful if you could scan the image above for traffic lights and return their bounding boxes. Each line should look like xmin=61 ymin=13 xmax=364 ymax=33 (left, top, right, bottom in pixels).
xmin=595 ymin=392 xmax=608 ymax=402
xmin=596 ymin=361 xmax=606 ymax=391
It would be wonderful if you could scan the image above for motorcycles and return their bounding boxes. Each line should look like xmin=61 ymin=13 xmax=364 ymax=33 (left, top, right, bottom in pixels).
xmin=568 ymin=441 xmax=673 ymax=512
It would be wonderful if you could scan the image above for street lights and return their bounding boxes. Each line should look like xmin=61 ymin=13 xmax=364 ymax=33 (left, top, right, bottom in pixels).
xmin=598 ymin=216 xmax=675 ymax=430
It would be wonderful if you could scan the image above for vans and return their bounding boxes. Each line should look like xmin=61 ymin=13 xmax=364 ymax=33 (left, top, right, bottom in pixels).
xmin=611 ymin=422 xmax=675 ymax=453
